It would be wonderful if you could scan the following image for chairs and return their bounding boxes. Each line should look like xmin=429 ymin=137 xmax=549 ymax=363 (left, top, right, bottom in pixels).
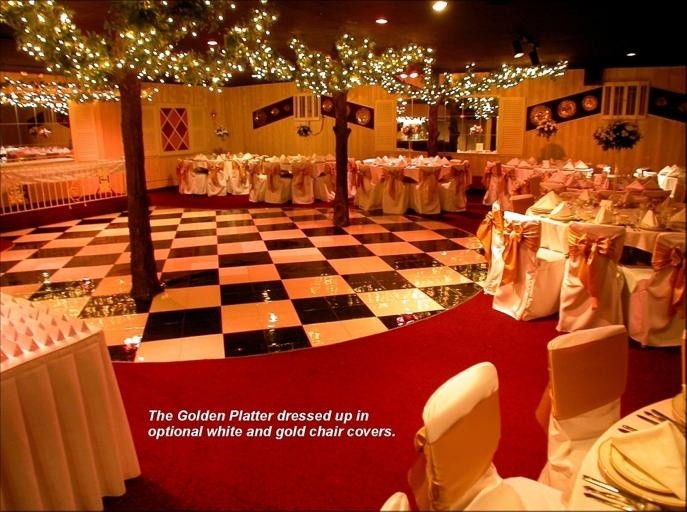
xmin=180 ymin=144 xmax=685 ymax=349
xmin=411 ymin=357 xmax=567 ymax=510
xmin=543 ymin=323 xmax=630 ymax=494
xmin=376 ymin=488 xmax=411 ymax=511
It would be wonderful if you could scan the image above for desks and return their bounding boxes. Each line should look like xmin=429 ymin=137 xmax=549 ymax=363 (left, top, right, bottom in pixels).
xmin=6 ymin=147 xmax=72 ymax=161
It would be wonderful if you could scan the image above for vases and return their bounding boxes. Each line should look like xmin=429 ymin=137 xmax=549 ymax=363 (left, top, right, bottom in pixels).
xmin=475 ymin=140 xmax=483 ymax=152
xmin=407 ymin=138 xmax=412 ymax=151
xmin=544 ymin=137 xmax=551 ymax=160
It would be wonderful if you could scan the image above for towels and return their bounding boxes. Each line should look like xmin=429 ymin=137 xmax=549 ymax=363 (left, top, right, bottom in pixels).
xmin=612 ymin=420 xmax=686 ymax=504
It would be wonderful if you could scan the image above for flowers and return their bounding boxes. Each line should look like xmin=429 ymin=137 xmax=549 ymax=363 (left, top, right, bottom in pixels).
xmin=215 ymin=124 xmax=230 ymax=142
xmin=467 ymin=126 xmax=483 ymax=141
xmin=402 ymin=125 xmax=416 ymax=139
xmin=594 ymin=118 xmax=641 ymax=150
xmin=536 ymin=119 xmax=558 ymax=141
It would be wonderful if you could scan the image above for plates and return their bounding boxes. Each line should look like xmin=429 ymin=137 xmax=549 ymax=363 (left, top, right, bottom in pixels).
xmin=596 ymin=434 xmax=687 ymax=507
xmin=530 ymin=208 xmax=686 ymax=235
